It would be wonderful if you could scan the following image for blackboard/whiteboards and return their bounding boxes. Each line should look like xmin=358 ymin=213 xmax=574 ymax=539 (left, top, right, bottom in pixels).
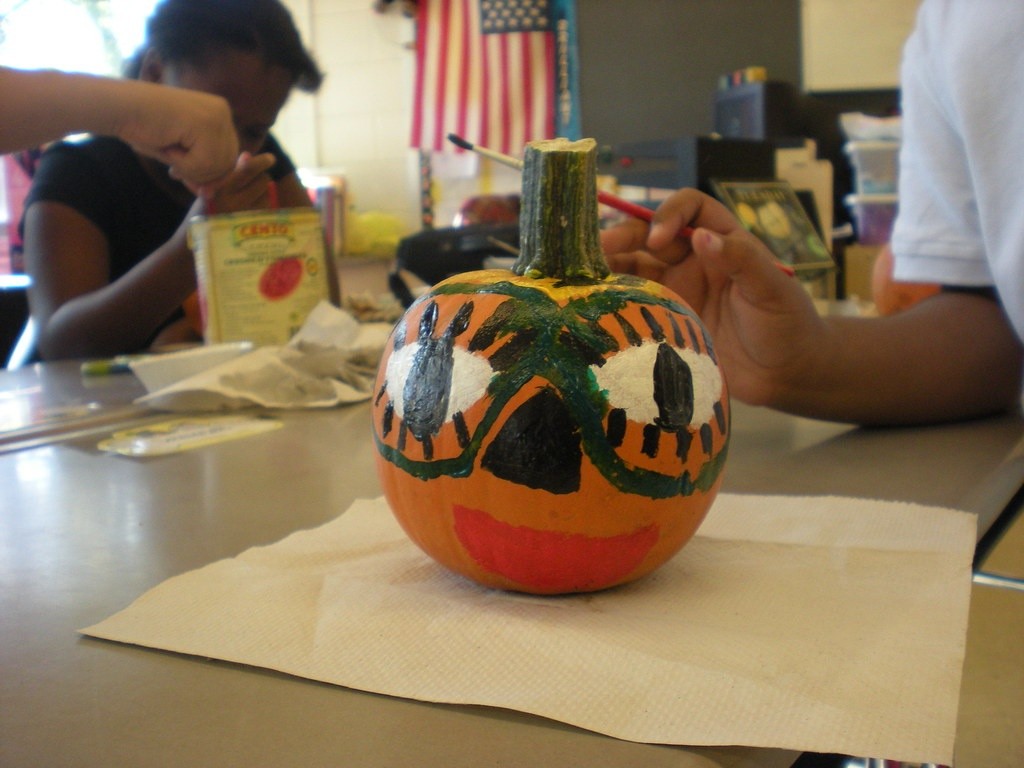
xmin=713 ymin=179 xmax=835 ymax=270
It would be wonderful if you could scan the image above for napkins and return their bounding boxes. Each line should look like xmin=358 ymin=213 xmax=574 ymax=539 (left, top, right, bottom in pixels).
xmin=135 ymin=300 xmax=395 ymax=413
xmin=79 ymin=494 xmax=980 ymax=768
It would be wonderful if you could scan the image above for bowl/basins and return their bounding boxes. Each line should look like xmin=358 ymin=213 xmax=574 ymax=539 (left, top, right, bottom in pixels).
xmin=843 ymin=140 xmax=899 ymax=246
xmin=126 ymin=342 xmax=251 ymax=394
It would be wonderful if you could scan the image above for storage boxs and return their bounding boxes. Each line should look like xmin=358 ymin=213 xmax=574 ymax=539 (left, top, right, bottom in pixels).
xmin=844 ymin=141 xmax=900 ymax=300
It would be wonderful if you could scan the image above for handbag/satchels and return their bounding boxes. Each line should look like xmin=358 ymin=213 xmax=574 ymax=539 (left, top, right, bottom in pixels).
xmin=387 ymin=225 xmax=520 ymax=306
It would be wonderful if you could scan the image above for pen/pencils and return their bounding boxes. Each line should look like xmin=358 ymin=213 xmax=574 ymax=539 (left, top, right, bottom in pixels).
xmin=81 ymin=360 xmax=132 ymax=376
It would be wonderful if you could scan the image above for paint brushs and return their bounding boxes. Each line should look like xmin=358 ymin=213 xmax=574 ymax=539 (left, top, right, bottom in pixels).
xmin=450 ymin=132 xmax=800 ymax=278
xmin=0 ymin=407 xmax=162 ymax=449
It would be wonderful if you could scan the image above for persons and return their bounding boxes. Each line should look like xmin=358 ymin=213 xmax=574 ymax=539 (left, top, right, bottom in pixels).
xmin=599 ymin=0 xmax=1024 ymax=426
xmin=0 ymin=67 xmax=239 ymax=188
xmin=6 ymin=0 xmax=342 ymax=372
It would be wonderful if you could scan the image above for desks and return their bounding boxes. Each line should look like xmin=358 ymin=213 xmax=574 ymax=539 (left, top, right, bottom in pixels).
xmin=0 ymin=299 xmax=1024 ymax=768
